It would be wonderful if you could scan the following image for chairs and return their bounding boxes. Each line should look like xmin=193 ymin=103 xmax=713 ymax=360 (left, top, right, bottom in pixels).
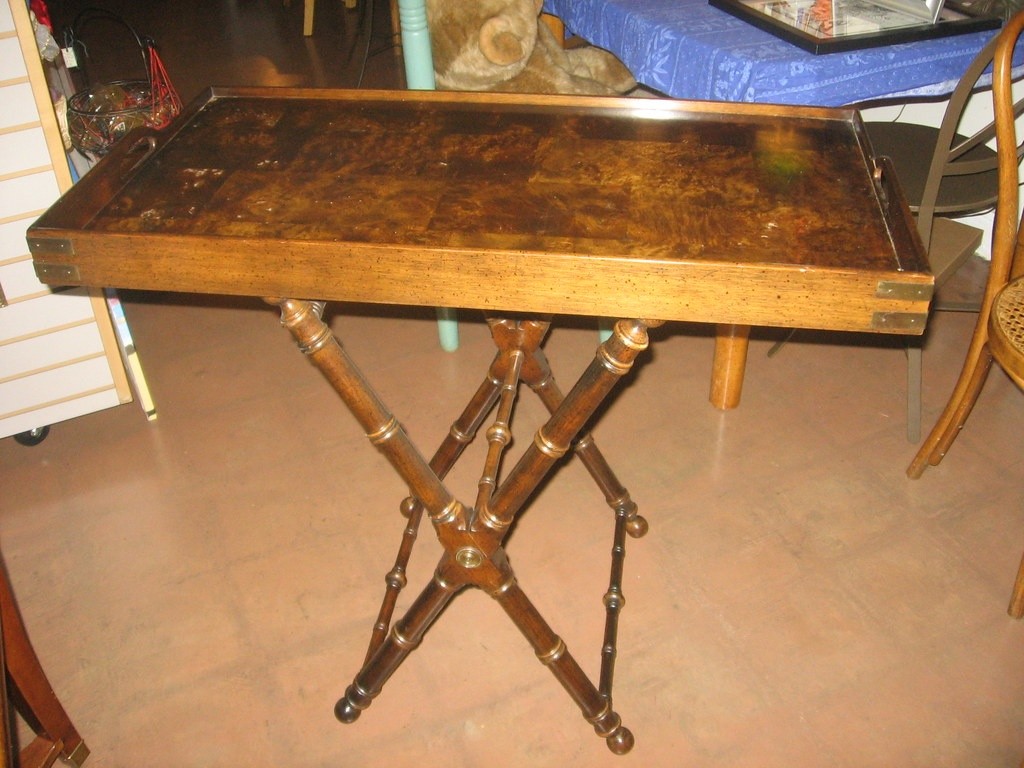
xmin=766 ymin=8 xmax=1024 ymax=618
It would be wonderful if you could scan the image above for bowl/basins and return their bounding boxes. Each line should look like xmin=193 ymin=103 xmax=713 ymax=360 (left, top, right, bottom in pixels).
xmin=67 ymin=80 xmax=180 ymax=152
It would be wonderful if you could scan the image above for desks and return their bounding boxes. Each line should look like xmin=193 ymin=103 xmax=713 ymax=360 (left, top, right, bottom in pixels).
xmin=540 ymin=0 xmax=1024 ymax=410
xmin=25 ymin=86 xmax=942 ymax=759
